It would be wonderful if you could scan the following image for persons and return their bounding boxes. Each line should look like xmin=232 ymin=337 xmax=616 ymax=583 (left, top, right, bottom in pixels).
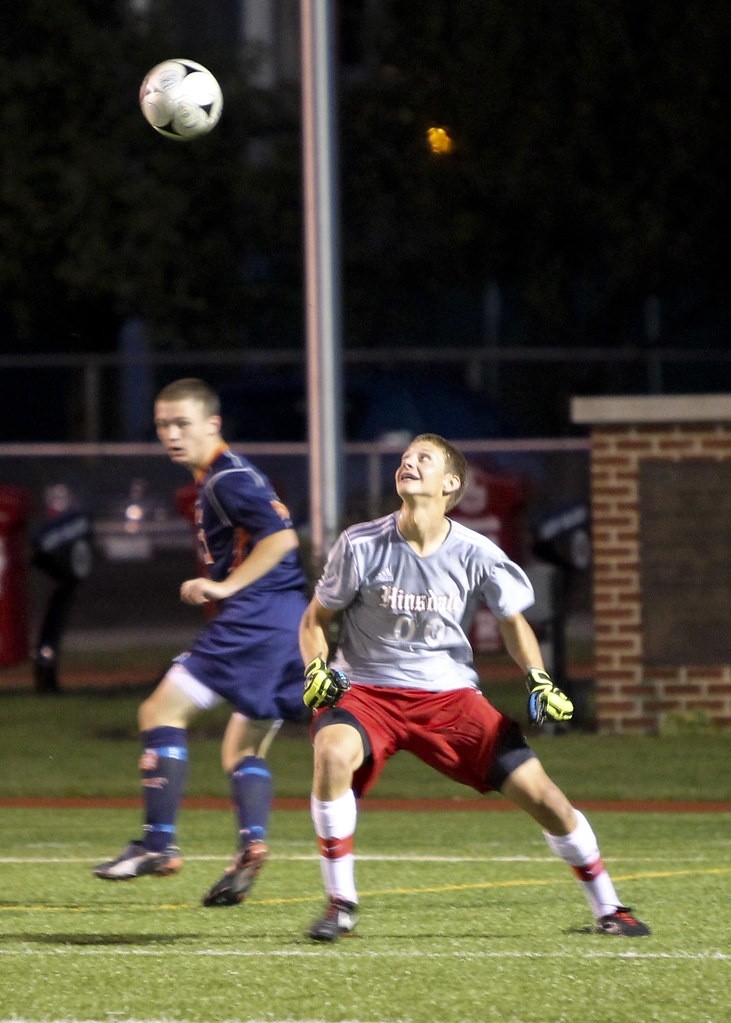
xmin=30 ymin=483 xmax=92 ymax=693
xmin=87 ymin=379 xmax=309 ymax=906
xmin=297 ymin=435 xmax=651 ymax=943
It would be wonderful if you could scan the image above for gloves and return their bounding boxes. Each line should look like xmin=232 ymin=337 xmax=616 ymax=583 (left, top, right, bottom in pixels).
xmin=524 ymin=669 xmax=574 ymax=726
xmin=302 ymin=657 xmax=350 ymax=710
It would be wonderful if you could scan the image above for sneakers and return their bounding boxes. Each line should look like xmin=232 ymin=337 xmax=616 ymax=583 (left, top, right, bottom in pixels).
xmin=595 ymin=907 xmax=652 ymax=936
xmin=92 ymin=841 xmax=184 ymax=879
xmin=204 ymin=850 xmax=268 ymax=906
xmin=309 ymin=896 xmax=358 ymax=940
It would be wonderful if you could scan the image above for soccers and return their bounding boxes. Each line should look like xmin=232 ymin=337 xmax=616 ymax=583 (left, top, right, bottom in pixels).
xmin=138 ymin=58 xmax=223 ymax=139
